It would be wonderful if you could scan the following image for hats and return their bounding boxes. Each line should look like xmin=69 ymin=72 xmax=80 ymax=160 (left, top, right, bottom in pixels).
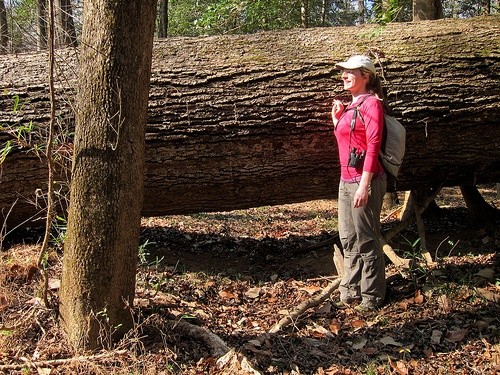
xmin=335 ymin=55 xmax=376 ymax=78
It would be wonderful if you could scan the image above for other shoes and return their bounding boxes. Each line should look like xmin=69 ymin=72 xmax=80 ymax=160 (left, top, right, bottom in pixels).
xmin=332 ymin=298 xmax=347 ymax=309
xmin=355 ymin=304 xmax=374 ymax=312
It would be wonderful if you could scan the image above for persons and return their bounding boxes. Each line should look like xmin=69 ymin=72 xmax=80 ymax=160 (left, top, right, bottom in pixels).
xmin=330 ymin=54 xmax=386 ymax=311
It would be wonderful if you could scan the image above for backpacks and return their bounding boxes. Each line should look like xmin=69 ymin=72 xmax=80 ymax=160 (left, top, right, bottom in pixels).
xmin=356 ymin=94 xmax=406 ymax=178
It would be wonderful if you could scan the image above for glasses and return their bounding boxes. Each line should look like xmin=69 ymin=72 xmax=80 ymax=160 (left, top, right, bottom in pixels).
xmin=339 ymin=70 xmax=361 ymax=76
